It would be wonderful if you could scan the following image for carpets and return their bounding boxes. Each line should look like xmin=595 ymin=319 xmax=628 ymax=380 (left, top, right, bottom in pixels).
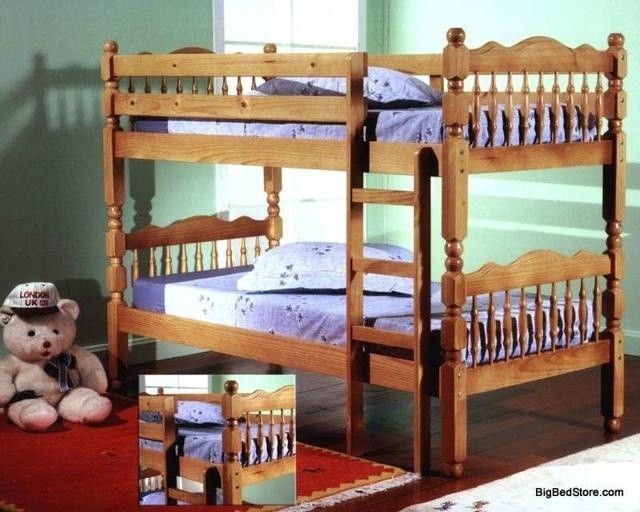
xmin=0 ymin=392 xmax=420 ymax=511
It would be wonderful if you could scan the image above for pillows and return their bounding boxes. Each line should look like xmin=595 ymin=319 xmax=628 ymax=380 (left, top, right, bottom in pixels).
xmin=237 ymin=242 xmax=415 ymax=298
xmin=140 ymin=400 xmax=225 ymax=425
xmin=254 ymin=66 xmax=443 ymax=109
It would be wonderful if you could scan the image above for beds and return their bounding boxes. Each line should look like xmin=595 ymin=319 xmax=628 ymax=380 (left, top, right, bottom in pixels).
xmin=139 ymin=381 xmax=296 ymax=504
xmin=101 ymin=28 xmax=627 ymax=476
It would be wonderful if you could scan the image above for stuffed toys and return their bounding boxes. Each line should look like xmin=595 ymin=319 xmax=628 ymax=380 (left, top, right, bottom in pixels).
xmin=0 ymin=298 xmax=112 ymax=433
